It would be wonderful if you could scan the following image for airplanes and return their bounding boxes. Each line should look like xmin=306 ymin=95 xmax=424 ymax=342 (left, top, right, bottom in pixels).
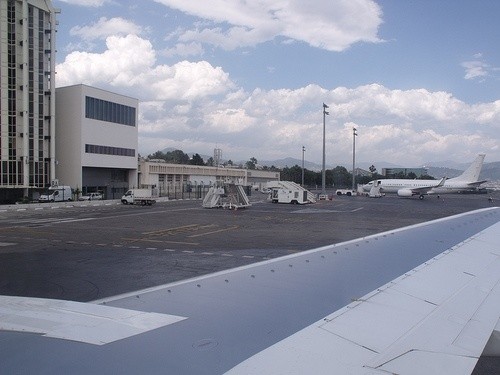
xmin=0 ymin=206 xmax=500 ymax=375
xmin=362 ymin=153 xmax=488 ymax=200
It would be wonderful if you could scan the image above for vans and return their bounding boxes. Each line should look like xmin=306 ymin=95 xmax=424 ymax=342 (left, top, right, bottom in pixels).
xmin=39 ymin=185 xmax=72 ymax=203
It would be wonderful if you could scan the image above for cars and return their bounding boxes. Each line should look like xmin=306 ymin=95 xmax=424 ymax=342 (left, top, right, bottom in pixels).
xmin=78 ymin=192 xmax=102 ymax=201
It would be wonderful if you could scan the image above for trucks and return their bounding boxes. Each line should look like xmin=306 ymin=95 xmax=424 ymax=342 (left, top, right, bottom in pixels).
xmin=121 ymin=188 xmax=158 ymax=205
xmin=272 ymin=188 xmax=310 ymax=205
